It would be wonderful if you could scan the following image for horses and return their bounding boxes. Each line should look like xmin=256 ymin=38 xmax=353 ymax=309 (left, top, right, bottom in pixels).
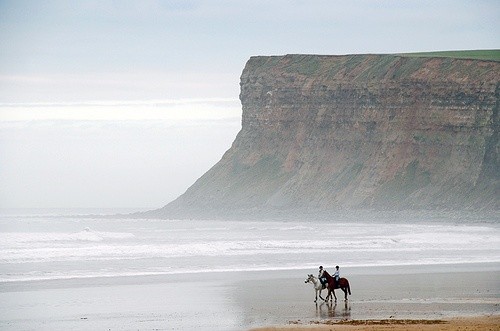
xmin=318 ymin=269 xmax=351 ymax=300
xmin=304 ymin=274 xmax=333 ymax=302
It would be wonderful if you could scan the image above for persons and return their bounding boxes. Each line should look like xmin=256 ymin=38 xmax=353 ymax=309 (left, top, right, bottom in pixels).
xmin=332 ymin=265 xmax=340 ymax=287
xmin=318 ymin=266 xmax=328 ymax=288
